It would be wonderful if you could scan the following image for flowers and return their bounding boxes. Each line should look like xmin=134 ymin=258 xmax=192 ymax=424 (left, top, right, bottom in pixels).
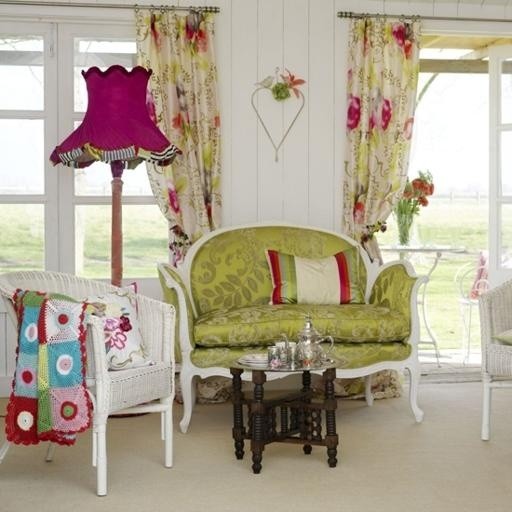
xmin=397 ymin=174 xmax=435 ymax=244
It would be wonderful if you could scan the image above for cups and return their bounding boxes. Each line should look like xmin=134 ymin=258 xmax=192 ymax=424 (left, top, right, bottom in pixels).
xmin=268 ymin=342 xmax=297 ymax=364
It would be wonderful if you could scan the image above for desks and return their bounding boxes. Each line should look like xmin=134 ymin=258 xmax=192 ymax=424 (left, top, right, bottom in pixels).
xmin=377 ymin=245 xmax=473 ymax=368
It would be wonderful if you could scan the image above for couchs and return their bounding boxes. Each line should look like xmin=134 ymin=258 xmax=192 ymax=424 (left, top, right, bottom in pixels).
xmin=158 ymin=222 xmax=431 ymax=435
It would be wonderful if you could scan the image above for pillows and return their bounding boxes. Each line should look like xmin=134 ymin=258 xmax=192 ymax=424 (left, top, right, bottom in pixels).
xmin=262 ymin=239 xmax=364 ymax=309
xmin=79 ymin=280 xmax=156 ymax=373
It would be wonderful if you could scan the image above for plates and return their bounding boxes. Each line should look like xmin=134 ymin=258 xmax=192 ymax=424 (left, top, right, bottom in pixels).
xmin=238 ymin=354 xmax=267 ymax=365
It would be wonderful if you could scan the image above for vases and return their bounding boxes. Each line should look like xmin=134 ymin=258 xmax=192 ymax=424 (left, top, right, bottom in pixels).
xmin=394 ymin=215 xmax=416 ymax=246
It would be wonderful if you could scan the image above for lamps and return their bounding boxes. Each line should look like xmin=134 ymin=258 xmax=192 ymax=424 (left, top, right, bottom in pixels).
xmin=50 ymin=65 xmax=184 ymax=282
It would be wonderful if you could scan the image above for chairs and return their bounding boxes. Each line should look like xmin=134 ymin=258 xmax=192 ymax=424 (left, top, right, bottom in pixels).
xmin=1 ymin=271 xmax=181 ymax=495
xmin=476 ymin=277 xmax=512 ymax=441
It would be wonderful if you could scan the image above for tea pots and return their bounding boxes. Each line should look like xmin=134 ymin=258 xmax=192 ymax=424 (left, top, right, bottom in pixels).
xmin=281 ymin=322 xmax=334 ymax=360
